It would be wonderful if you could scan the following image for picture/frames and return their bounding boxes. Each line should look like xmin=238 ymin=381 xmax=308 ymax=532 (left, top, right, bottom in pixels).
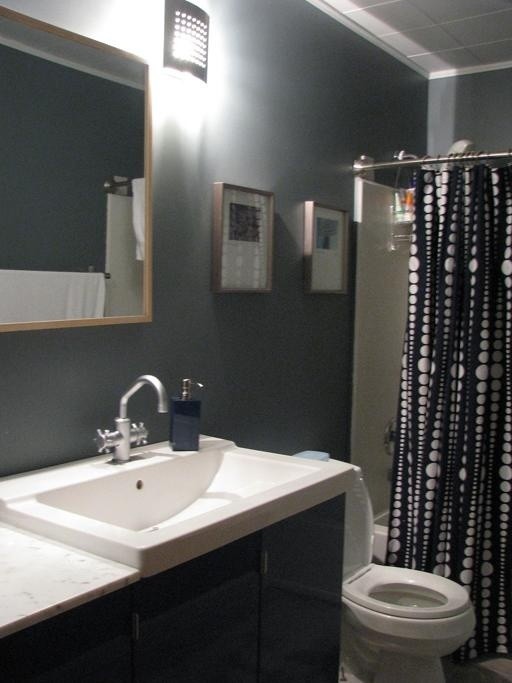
xmin=302 ymin=201 xmax=351 ymax=294
xmin=214 ymin=181 xmax=274 ymax=295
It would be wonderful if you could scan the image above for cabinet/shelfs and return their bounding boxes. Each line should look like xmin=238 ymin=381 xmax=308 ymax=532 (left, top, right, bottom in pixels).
xmin=0 ymin=493 xmax=348 ymax=683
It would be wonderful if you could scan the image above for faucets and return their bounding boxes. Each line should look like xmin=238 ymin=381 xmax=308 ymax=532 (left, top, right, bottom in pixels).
xmin=113 ymin=372 xmax=168 ymax=459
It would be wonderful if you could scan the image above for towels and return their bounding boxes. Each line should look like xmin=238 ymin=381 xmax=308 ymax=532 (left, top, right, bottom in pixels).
xmin=0 ymin=268 xmax=107 ymax=324
xmin=131 ymin=176 xmax=147 ymax=263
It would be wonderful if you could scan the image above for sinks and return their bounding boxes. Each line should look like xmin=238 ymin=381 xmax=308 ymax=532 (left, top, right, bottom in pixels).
xmin=0 ymin=434 xmax=354 ymax=573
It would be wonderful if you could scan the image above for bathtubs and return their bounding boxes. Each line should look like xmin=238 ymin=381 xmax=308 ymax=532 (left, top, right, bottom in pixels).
xmin=371 ymin=505 xmax=391 ymax=563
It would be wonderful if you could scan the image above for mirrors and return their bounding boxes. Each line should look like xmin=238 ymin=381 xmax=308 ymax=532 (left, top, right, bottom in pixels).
xmin=0 ymin=8 xmax=153 ymax=335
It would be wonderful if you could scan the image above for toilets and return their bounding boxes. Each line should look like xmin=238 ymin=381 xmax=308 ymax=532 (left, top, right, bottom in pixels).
xmin=292 ymin=447 xmax=478 ymax=682
